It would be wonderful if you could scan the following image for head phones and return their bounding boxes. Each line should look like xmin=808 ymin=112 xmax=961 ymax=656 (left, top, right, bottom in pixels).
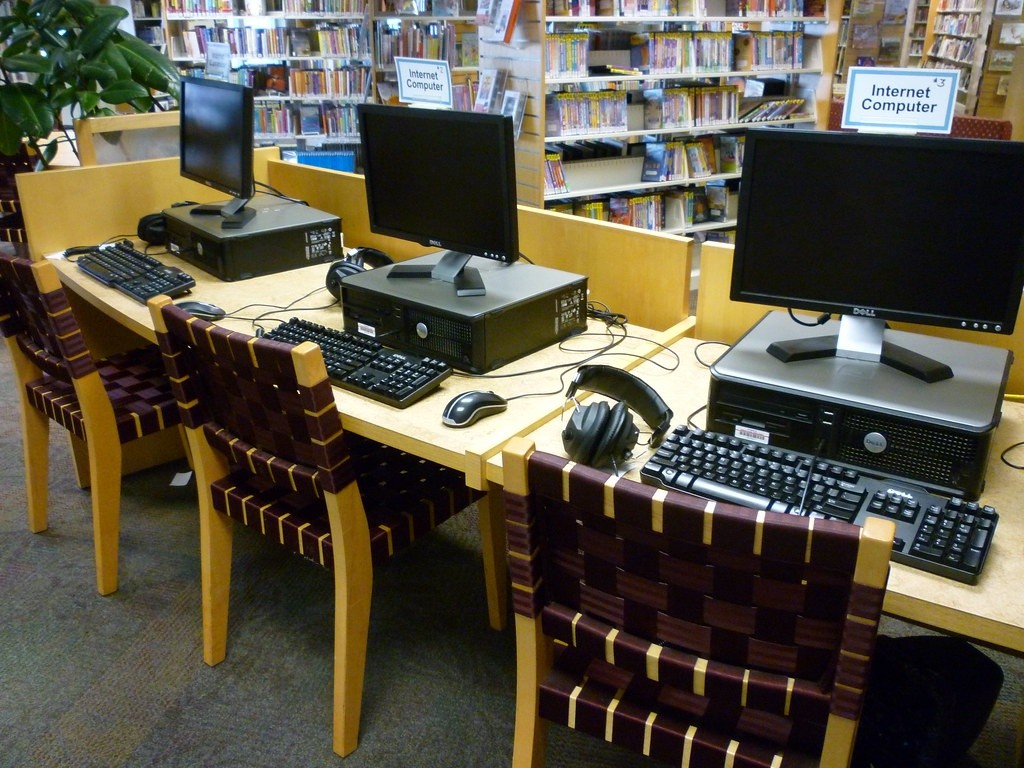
xmin=562 ymin=364 xmax=674 ymax=468
xmin=325 ymin=247 xmax=395 ymax=301
xmin=137 ymin=201 xmax=200 ymax=246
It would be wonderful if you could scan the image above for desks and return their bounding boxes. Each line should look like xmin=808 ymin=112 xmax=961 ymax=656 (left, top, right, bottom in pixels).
xmin=0 ymin=144 xmax=1024 ymax=768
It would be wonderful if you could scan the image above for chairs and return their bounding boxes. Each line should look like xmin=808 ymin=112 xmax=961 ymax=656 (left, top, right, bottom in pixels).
xmin=146 ymin=296 xmax=508 ymax=754
xmin=0 ymin=143 xmax=35 ymax=242
xmin=0 ymin=254 xmax=205 ymax=597
xmin=501 ymin=437 xmax=897 ymax=768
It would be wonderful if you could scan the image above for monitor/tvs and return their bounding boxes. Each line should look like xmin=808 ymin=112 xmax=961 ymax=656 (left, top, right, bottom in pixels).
xmin=730 ymin=128 xmax=1024 ymax=383
xmin=357 ymin=103 xmax=520 ymax=297
xmin=178 ymin=75 xmax=257 ymax=229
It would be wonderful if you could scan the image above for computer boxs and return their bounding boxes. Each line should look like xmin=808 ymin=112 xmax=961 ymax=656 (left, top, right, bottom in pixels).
xmin=341 ymin=250 xmax=588 ymax=375
xmin=161 ymin=194 xmax=346 ymax=283
xmin=704 ymin=310 xmax=1015 ymax=500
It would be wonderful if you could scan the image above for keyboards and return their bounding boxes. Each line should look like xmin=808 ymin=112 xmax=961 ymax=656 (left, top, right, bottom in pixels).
xmin=264 ymin=317 xmax=453 ymax=409
xmin=641 ymin=425 xmax=1000 ymax=587
xmin=77 ymin=242 xmax=196 ymax=305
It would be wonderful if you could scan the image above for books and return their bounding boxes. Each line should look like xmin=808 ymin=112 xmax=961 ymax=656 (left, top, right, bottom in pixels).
xmin=545 ymin=131 xmax=746 ymax=195
xmin=544 ymin=185 xmax=736 ymax=245
xmin=170 ymin=66 xmax=373 ymax=98
xmin=130 ymin=0 xmax=466 ymax=19
xmin=474 ymin=0 xmax=522 ymax=44
xmin=833 ymin=0 xmax=911 ymax=84
xmin=545 ymin=0 xmax=827 ymax=17
xmin=281 ymin=142 xmax=363 ymax=174
xmin=136 ymin=22 xmax=479 ymax=68
xmin=375 ymin=69 xmax=526 ymax=142
xmin=253 ymin=102 xmax=360 ymax=135
xmin=545 ymin=21 xmax=805 ymax=80
xmin=545 ymin=76 xmax=817 ymax=137
xmin=909 ymin=0 xmax=1024 ymax=113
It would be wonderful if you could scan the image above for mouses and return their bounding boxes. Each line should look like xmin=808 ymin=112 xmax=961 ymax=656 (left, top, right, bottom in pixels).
xmin=174 ymin=301 xmax=226 ymax=322
xmin=441 ymin=389 xmax=507 ymax=428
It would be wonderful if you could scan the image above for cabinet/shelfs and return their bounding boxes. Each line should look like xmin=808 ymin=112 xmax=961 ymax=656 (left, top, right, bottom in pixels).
xmin=834 ymin=0 xmax=907 ymax=85
xmin=165 ymin=0 xmax=478 ymax=165
xmin=109 ymin=0 xmax=174 ymax=113
xmin=920 ymin=0 xmax=1024 ymax=120
xmin=899 ymin=0 xmax=930 ymax=68
xmin=476 ymin=0 xmax=839 ymax=293
